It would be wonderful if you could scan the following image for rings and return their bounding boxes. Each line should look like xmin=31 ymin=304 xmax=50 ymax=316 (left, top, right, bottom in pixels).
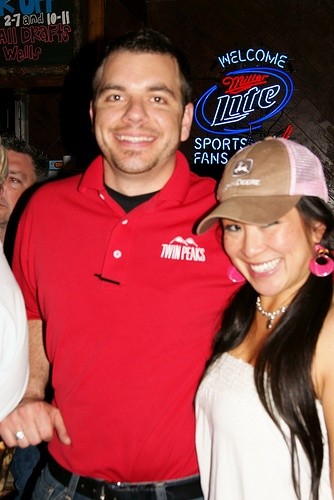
xmin=15 ymin=431 xmax=25 ymax=440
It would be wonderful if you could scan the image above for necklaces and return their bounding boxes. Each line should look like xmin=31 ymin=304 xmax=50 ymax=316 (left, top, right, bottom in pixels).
xmin=256 ymin=297 xmax=287 ymax=328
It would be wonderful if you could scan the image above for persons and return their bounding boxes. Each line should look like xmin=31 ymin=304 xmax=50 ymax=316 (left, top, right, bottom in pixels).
xmin=195 ymin=139 xmax=334 ymax=500
xmin=0 ymin=138 xmax=54 ymax=500
xmin=0 ymin=25 xmax=245 ymax=500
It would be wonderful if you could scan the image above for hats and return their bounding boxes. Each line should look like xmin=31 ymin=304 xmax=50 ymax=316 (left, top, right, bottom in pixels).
xmin=195 ymin=136 xmax=328 ymax=233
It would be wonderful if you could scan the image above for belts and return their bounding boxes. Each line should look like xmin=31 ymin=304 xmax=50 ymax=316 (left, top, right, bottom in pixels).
xmin=47 ymin=457 xmax=203 ymax=500
